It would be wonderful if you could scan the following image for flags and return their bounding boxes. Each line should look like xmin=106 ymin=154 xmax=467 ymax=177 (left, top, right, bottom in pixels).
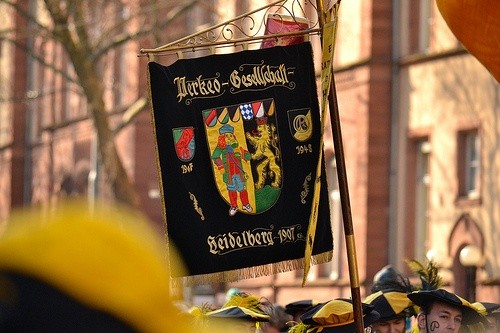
xmin=146 ymin=40 xmax=334 ymax=292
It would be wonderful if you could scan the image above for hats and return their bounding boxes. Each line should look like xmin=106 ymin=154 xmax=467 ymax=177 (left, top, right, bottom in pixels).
xmin=363 ymin=288 xmax=415 ymax=321
xmin=472 ymin=301 xmax=500 ymax=330
xmin=402 ymin=288 xmax=485 ymax=324
xmin=285 ymin=299 xmax=319 ymax=315
xmin=202 ymin=294 xmax=271 ymax=322
xmin=286 ymin=298 xmax=380 ymax=333
xmin=0 ymin=202 xmax=243 ymax=333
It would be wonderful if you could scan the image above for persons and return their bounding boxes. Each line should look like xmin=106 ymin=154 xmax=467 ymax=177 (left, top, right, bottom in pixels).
xmin=182 ymin=256 xmax=500 ymax=333
